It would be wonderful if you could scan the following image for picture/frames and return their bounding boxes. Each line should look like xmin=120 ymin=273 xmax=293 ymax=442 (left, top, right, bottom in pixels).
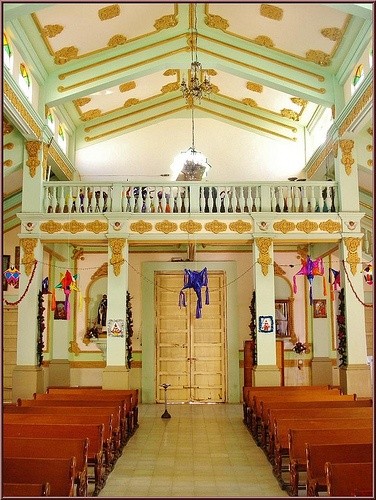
xmin=257 ymin=316 xmax=274 ymax=333
xmin=276 ymin=300 xmax=291 ymax=339
xmin=53 ymin=301 xmax=68 ymax=321
xmin=102 ymin=297 xmax=107 ymax=326
xmin=313 ymin=298 xmax=328 ymax=318
xmin=107 ymin=319 xmax=124 ymax=338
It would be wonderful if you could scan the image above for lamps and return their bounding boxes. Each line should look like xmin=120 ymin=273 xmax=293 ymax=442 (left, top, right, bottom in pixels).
xmin=177 ymin=60 xmax=213 ymax=108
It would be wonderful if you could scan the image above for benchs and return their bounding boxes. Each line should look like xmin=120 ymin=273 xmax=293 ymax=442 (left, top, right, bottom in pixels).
xmin=1 ymin=387 xmax=140 ymax=498
xmin=239 ymin=383 xmax=374 ymax=497
